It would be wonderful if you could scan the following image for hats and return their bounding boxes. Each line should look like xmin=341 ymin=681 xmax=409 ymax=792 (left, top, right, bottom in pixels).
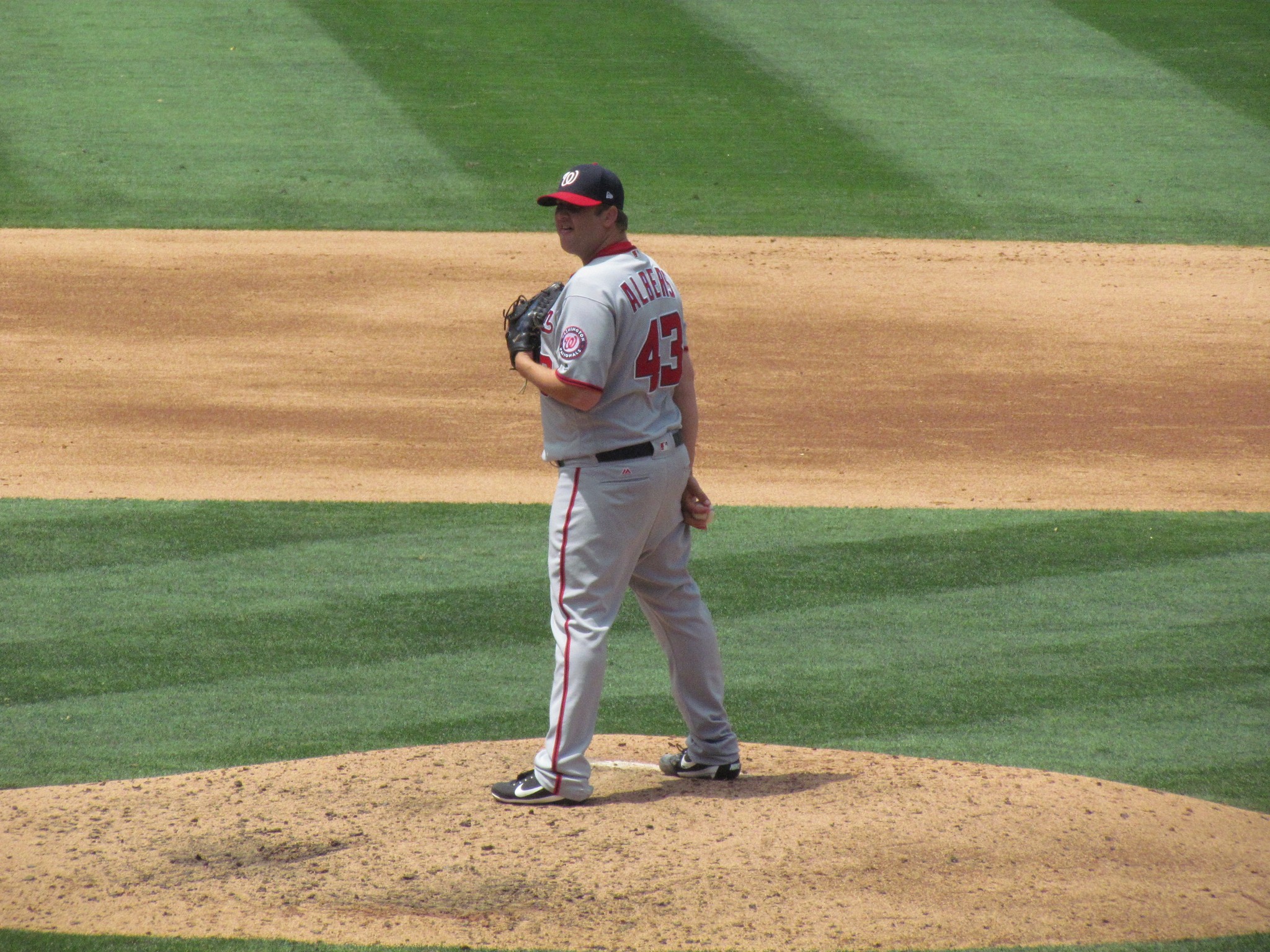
xmin=536 ymin=163 xmax=625 ymax=210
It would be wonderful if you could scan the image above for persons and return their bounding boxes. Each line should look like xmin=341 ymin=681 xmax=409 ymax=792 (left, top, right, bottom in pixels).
xmin=490 ymin=163 xmax=742 ymax=805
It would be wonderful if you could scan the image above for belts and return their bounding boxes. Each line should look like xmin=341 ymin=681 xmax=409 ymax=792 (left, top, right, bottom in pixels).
xmin=558 ymin=431 xmax=687 ymax=466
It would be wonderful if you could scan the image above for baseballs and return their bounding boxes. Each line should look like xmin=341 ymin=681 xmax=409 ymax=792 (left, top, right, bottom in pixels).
xmin=692 ymin=499 xmax=714 ymax=523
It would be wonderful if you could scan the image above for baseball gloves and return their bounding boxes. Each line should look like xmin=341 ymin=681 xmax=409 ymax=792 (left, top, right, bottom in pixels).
xmin=505 ymin=281 xmax=564 ymax=369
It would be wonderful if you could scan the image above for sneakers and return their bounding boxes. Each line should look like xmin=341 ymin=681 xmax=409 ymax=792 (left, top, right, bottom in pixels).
xmin=660 ymin=743 xmax=741 ymax=781
xmin=491 ymin=769 xmax=590 ymax=804
